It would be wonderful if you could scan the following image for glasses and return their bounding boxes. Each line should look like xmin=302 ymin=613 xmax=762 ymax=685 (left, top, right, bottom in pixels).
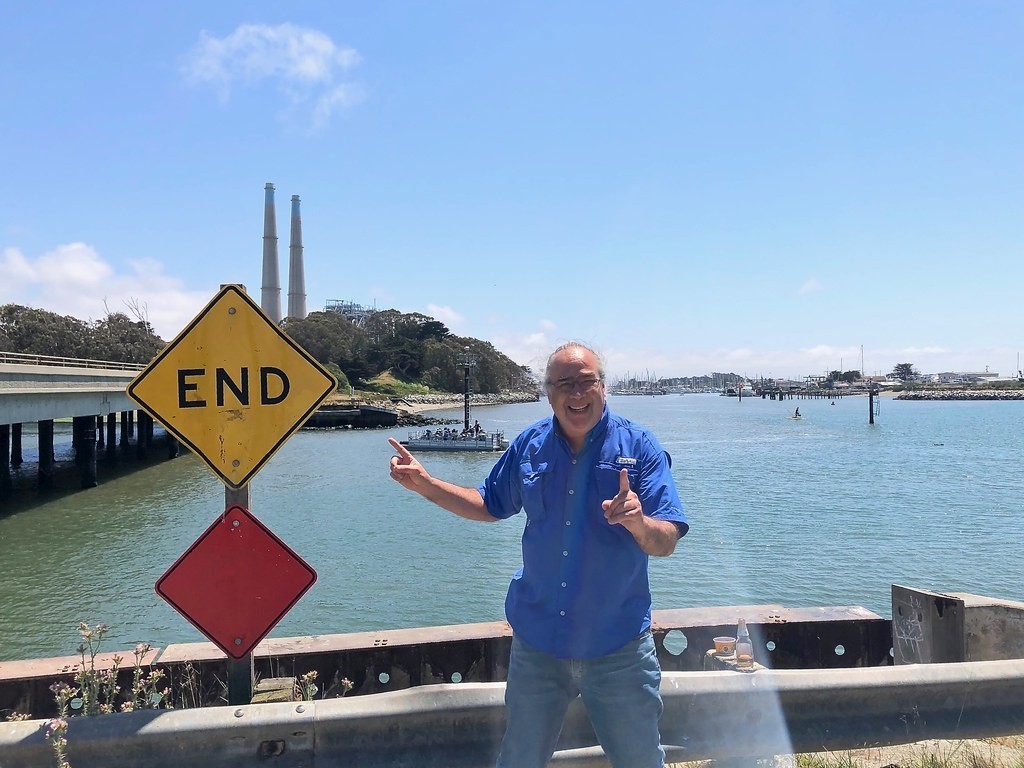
xmin=547 ymin=377 xmax=601 ymax=393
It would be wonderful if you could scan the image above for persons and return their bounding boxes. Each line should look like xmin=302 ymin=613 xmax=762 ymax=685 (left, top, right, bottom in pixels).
xmin=388 ymin=340 xmax=690 ymax=768
xmin=795 ymin=407 xmax=801 ymax=417
xmin=418 ymin=419 xmax=487 ymax=443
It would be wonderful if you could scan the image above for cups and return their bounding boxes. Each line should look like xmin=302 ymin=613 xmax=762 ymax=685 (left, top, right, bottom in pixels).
xmin=713 ymin=635 xmax=737 ymax=655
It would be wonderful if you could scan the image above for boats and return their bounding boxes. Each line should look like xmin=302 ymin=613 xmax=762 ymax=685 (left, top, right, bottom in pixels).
xmin=399 ymin=430 xmax=509 ymax=452
xmin=735 ymin=380 xmax=756 ymax=397
xmin=720 ymin=382 xmax=737 ymax=397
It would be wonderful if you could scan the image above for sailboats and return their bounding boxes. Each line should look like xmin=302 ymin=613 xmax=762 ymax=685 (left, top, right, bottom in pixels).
xmin=606 ymin=369 xmax=723 ymax=396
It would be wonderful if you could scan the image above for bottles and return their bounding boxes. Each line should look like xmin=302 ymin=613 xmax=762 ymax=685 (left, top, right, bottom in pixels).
xmin=736 ymin=615 xmax=755 ymax=669
